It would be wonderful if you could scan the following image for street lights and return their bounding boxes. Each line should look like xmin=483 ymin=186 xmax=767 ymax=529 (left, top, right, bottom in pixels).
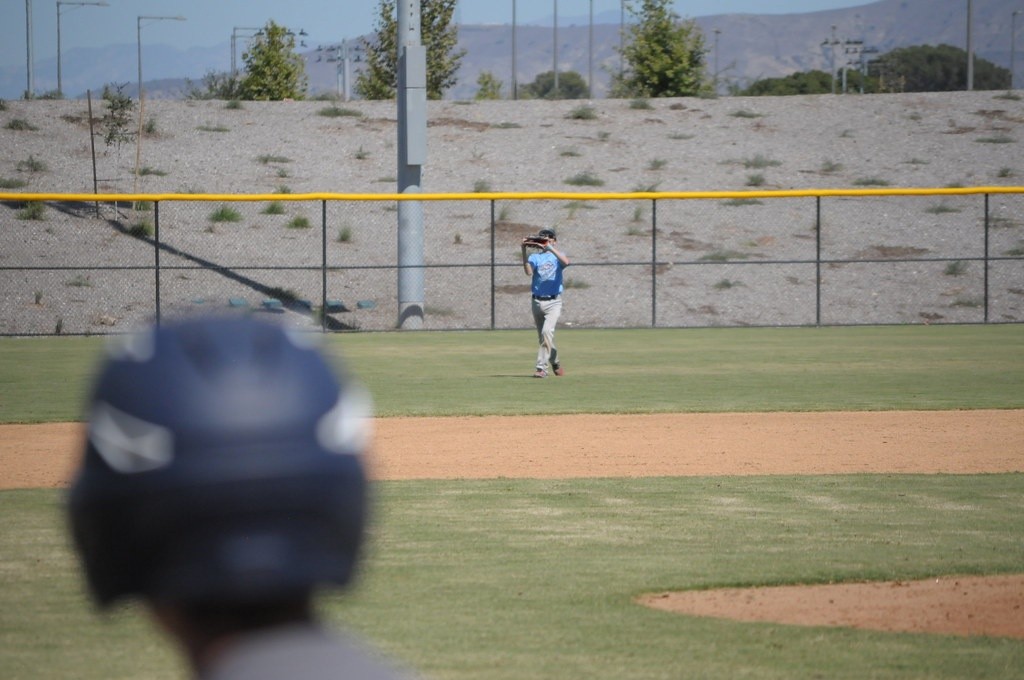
xmin=57 ymin=2 xmax=112 ymax=96
xmin=138 ymin=15 xmax=189 ymax=101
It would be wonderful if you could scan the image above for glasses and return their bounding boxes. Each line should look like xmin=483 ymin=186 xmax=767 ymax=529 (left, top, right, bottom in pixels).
xmin=541 ymin=235 xmax=554 ymax=238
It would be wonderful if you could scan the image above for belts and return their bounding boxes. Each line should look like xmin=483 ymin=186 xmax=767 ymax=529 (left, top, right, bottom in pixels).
xmin=532 ymin=294 xmax=558 ymax=300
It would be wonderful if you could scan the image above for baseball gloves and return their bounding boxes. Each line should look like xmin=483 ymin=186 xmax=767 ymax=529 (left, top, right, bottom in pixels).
xmin=523 ymin=235 xmax=550 ymax=250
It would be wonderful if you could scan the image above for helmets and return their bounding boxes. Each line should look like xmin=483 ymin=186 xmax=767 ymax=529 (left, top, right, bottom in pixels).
xmin=69 ymin=316 xmax=363 ymax=606
xmin=539 ymin=228 xmax=556 ymax=242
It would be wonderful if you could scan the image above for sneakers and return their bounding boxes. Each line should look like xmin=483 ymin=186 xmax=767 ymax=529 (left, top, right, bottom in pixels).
xmin=535 ymin=368 xmax=547 ymax=377
xmin=552 ymin=363 xmax=562 ymax=376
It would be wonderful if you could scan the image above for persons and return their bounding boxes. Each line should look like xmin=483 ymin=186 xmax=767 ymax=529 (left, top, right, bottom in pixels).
xmin=62 ymin=299 xmax=421 ymax=680
xmin=521 ymin=226 xmax=570 ymax=378
